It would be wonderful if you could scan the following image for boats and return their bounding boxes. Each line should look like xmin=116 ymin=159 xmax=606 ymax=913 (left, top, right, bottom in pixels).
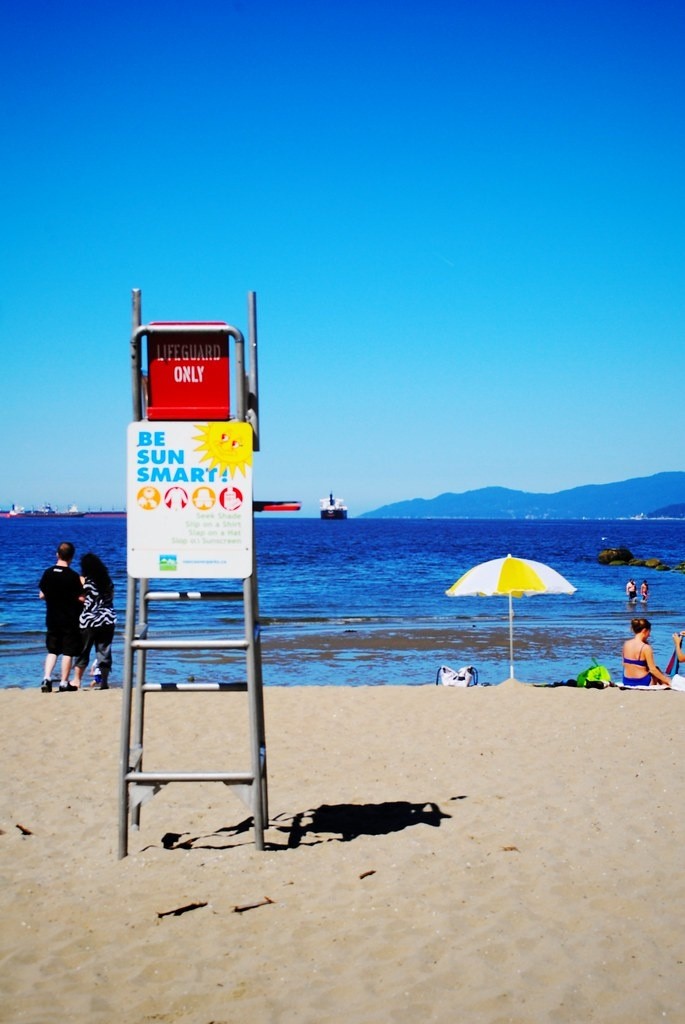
xmin=6 ymin=504 xmax=87 ymax=519
xmin=319 ymin=494 xmax=347 ymax=520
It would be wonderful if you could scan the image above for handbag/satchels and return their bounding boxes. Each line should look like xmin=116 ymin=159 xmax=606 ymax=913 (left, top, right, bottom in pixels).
xmin=435 ymin=665 xmax=478 ymax=687
xmin=577 ymin=657 xmax=611 ymax=688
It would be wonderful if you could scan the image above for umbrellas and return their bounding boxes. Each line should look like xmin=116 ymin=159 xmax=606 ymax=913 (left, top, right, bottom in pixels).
xmin=445 ymin=554 xmax=577 ymax=678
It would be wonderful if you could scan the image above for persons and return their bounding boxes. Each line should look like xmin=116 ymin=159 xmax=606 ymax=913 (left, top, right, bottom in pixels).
xmin=674 ymin=631 xmax=685 ymax=662
xmin=38 ymin=543 xmax=86 ymax=693
xmin=626 ymin=579 xmax=637 ymax=602
xmin=623 ymin=619 xmax=672 ymax=686
xmin=641 ymin=580 xmax=648 ymax=601
xmin=90 ymin=659 xmax=112 ymax=687
xmin=69 ymin=552 xmax=116 ymax=690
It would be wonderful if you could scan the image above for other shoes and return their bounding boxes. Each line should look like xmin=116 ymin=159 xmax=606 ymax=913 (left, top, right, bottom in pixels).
xmin=41 ymin=679 xmax=52 ymax=692
xmin=58 ymin=681 xmax=78 ymax=692
xmin=94 ymin=684 xmax=109 ymax=690
